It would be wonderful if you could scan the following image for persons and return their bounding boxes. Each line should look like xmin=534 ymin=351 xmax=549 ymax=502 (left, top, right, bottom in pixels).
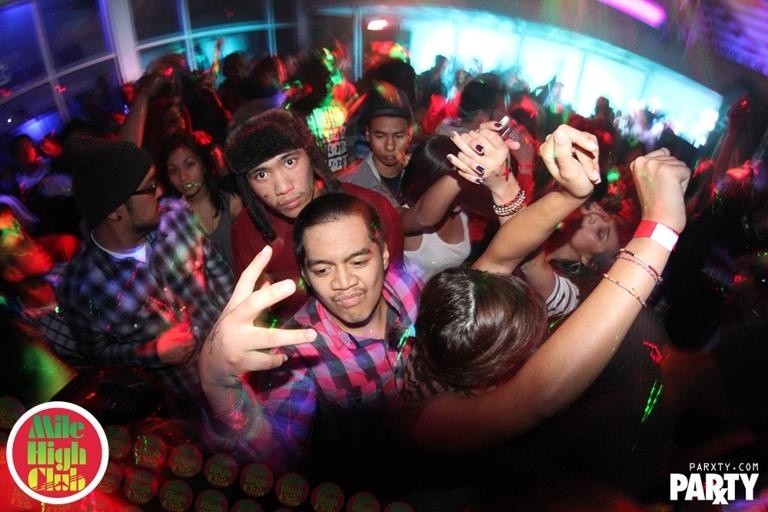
xmin=1 ymin=21 xmax=768 ymax=510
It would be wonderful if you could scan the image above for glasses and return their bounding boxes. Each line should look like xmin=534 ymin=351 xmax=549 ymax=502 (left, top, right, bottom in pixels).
xmin=133 ymin=184 xmax=158 ymax=195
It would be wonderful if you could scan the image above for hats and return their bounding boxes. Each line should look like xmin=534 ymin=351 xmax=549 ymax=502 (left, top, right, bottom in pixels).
xmin=72 ymin=141 xmax=152 ymax=230
xmin=367 ymin=97 xmax=411 ymax=121
xmin=223 ymin=108 xmax=341 ymax=243
